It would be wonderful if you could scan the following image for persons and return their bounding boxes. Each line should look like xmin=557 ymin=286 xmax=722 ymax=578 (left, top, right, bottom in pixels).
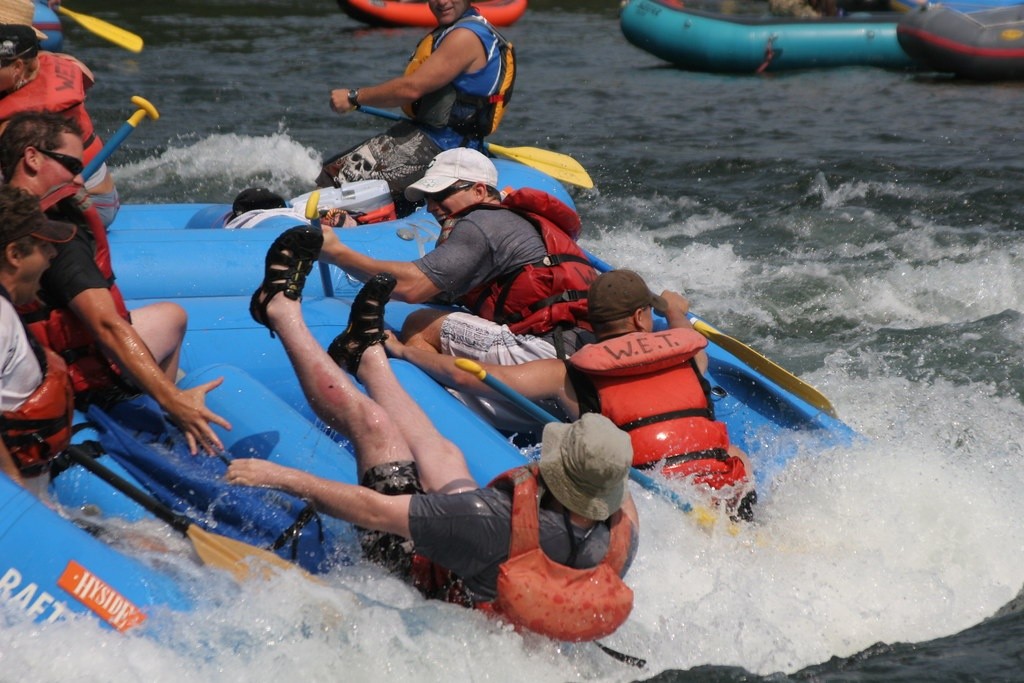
xmin=317 ymin=148 xmax=750 ymax=523
xmin=225 ymin=225 xmax=640 ymax=610
xmin=314 ymin=0 xmax=504 ymax=191
xmin=0 ymin=0 xmax=230 ymax=490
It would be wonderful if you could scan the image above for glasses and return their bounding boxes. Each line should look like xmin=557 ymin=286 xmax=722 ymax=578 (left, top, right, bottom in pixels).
xmin=34 ymin=147 xmax=83 ymax=175
xmin=0 ymin=46 xmax=35 ymax=68
xmin=423 ymin=184 xmax=492 ymax=205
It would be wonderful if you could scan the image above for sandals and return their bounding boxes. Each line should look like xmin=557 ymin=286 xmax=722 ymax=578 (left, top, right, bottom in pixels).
xmin=326 ymin=272 xmax=397 ymax=379
xmin=249 ymin=225 xmax=324 ymax=338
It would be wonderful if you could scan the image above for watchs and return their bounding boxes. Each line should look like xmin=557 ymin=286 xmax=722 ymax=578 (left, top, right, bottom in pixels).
xmin=348 ymin=88 xmax=361 ymax=108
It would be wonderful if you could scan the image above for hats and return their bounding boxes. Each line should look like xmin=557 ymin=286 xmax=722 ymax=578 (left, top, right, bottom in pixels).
xmin=539 ymin=412 xmax=634 ymax=521
xmin=403 ymin=146 xmax=498 ymax=203
xmin=0 ymin=0 xmax=49 ymax=41
xmin=0 ymin=206 xmax=76 ymax=244
xmin=587 ymin=269 xmax=668 ymax=323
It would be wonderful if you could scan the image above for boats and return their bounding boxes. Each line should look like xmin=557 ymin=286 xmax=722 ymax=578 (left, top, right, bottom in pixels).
xmin=0 ymin=201 xmax=864 ymax=668
xmin=619 ymin=0 xmax=935 ymax=74
xmin=339 ymin=0 xmax=531 ymax=25
xmin=886 ymin=0 xmax=1024 ymax=18
xmin=895 ymin=1 xmax=1024 ymax=80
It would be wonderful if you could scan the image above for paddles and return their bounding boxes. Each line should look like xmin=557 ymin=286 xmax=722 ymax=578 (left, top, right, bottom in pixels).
xmin=358 ymin=104 xmax=596 ymax=191
xmin=41 ymin=0 xmax=145 ymax=55
xmin=455 ymin=355 xmax=761 ymax=546
xmin=304 ymin=189 xmax=335 ymax=296
xmin=69 ymin=444 xmax=363 ymax=626
xmin=581 ymin=247 xmax=837 ymax=418
xmin=83 ymin=95 xmax=161 ymax=180
